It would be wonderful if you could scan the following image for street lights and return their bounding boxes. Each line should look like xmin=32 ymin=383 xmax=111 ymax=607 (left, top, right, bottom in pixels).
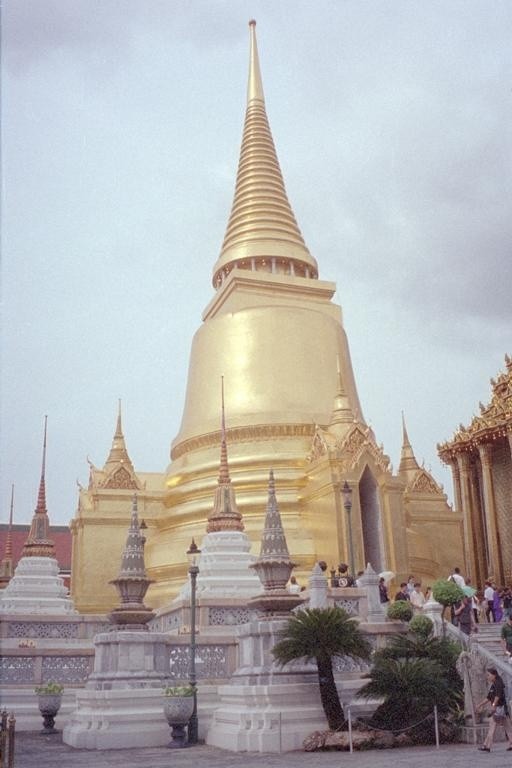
xmin=140 ymin=517 xmax=148 ymax=554
xmin=181 ymin=533 xmax=204 ymax=743
xmin=340 ymin=478 xmax=360 ymax=587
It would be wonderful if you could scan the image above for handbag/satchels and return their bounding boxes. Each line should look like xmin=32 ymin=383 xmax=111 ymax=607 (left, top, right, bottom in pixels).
xmin=492 ymin=705 xmax=505 ymax=719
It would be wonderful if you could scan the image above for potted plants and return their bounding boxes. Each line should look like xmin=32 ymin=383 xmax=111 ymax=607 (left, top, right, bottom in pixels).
xmin=34 ymin=679 xmax=64 ymax=735
xmin=160 ymin=686 xmax=198 ymax=749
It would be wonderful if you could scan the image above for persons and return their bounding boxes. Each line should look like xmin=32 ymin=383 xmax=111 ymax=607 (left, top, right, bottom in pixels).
xmin=474 ymin=667 xmax=512 ymax=751
xmin=289 ymin=559 xmax=512 ymax=638
xmin=501 ymin=613 xmax=512 ymax=664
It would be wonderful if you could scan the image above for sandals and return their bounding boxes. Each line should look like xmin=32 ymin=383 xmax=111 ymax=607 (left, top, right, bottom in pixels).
xmin=478 ymin=745 xmax=490 ymax=751
